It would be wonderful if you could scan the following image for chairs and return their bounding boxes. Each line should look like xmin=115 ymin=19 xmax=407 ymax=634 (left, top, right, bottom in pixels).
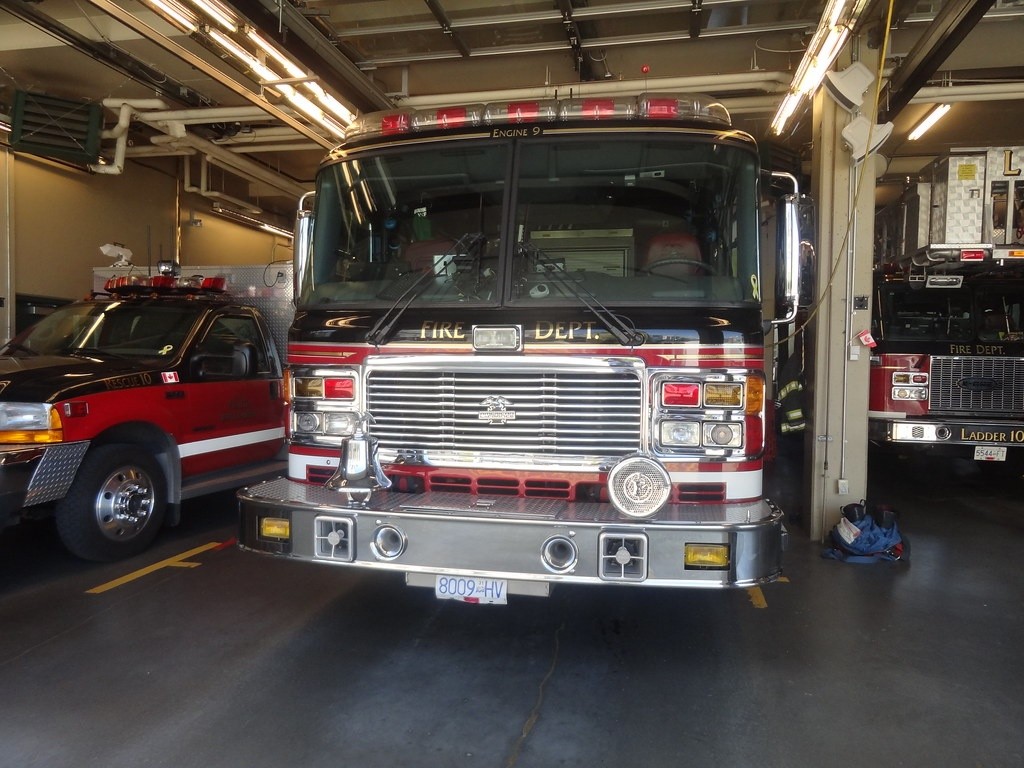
xmin=406 ymin=239 xmax=461 ymax=272
xmin=640 ymin=231 xmax=704 ymax=274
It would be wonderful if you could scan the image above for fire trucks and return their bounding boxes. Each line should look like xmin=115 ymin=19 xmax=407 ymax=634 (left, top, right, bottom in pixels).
xmin=865 ymin=245 xmax=1023 ymax=461
xmin=231 ymin=93 xmax=819 ymax=605
xmin=0 ymin=272 xmax=284 ymax=569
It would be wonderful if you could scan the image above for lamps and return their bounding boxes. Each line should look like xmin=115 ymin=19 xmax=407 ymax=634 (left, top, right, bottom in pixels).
xmin=140 ymin=0 xmax=358 ymax=141
xmin=842 ymin=114 xmax=894 ymax=167
xmin=766 ymin=0 xmax=878 ymax=137
xmin=822 ymin=64 xmax=876 ymax=114
xmin=907 ymin=102 xmax=951 ymax=141
